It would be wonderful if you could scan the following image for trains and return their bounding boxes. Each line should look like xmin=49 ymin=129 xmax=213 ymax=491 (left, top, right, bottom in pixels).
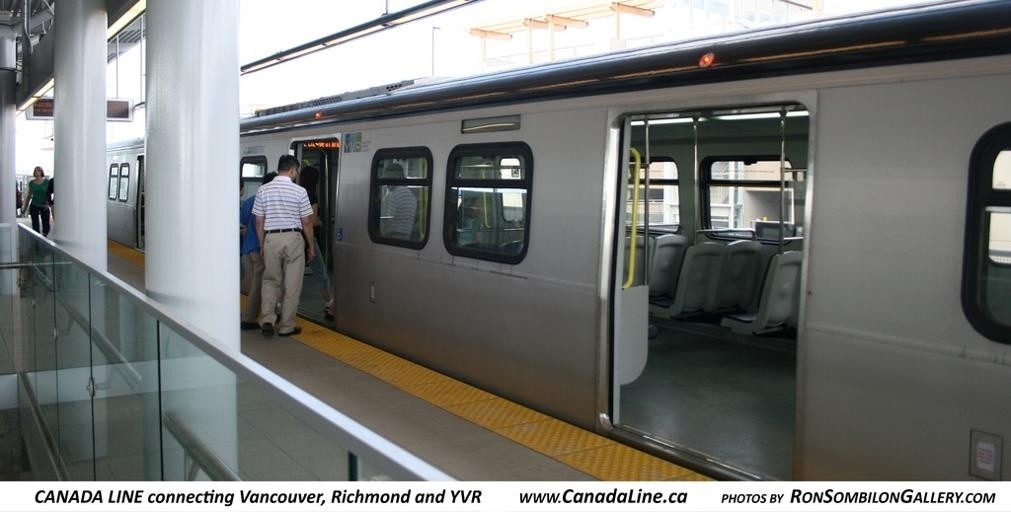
xmin=103 ymin=0 xmax=1011 ymax=482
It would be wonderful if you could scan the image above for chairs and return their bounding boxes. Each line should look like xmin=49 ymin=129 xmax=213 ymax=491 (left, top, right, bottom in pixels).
xmin=623 ymin=233 xmax=801 ymax=344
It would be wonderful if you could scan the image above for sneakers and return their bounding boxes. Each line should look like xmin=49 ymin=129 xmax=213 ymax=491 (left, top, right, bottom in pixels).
xmin=263 ymin=322 xmax=274 ymax=336
xmin=279 ymin=327 xmax=300 ymax=336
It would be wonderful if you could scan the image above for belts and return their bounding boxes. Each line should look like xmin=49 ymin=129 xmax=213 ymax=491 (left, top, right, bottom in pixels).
xmin=265 ymin=227 xmax=301 ymax=233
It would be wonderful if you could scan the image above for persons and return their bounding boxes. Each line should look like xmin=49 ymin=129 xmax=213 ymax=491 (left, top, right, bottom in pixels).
xmin=16 ymin=180 xmax=23 ymax=217
xmin=251 ymin=153 xmax=317 ymax=337
xmin=296 ymin=166 xmax=337 ymax=322
xmin=241 ymin=170 xmax=280 ymax=330
xmin=454 ymin=191 xmax=487 ymax=246
xmin=380 ymin=161 xmax=420 ymax=242
xmin=20 ymin=165 xmax=51 ymax=237
xmin=46 ymin=176 xmax=54 ymax=221
